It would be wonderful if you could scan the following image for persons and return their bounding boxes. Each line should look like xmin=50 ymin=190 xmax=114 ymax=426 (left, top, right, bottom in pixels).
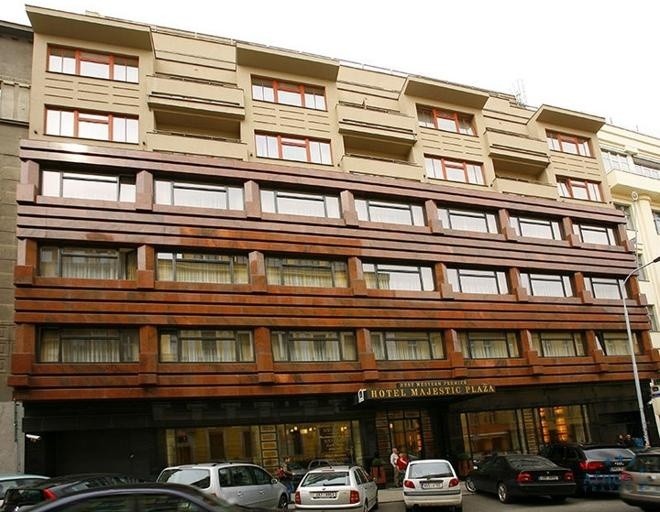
xmin=389 ymin=448 xmax=399 ymax=488
xmin=616 ymin=434 xmax=624 ymax=446
xmin=274 ymin=455 xmax=295 ymax=502
xmin=397 ymin=453 xmax=408 ymax=487
xmin=623 ymin=435 xmax=637 ymax=448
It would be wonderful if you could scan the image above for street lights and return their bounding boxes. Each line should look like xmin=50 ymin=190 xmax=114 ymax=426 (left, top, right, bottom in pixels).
xmin=619 ymin=255 xmax=660 ymax=448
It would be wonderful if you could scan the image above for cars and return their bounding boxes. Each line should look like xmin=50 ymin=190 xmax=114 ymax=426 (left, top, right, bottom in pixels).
xmin=400 ymin=458 xmax=464 ymax=512
xmin=1 ymin=469 xmax=165 ymax=512
xmin=307 ymin=459 xmax=340 ymax=470
xmin=284 ymin=462 xmax=305 ymax=483
xmin=294 ymin=464 xmax=380 ymax=512
xmin=464 ymin=453 xmax=578 ymax=506
xmin=12 ymin=481 xmax=298 ymax=512
xmin=0 ymin=472 xmax=51 ymax=510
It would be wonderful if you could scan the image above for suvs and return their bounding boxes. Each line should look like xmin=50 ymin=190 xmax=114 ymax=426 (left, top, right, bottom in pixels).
xmin=153 ymin=462 xmax=291 ymax=511
xmin=536 ymin=440 xmax=639 ymax=497
xmin=619 ymin=445 xmax=660 ymax=512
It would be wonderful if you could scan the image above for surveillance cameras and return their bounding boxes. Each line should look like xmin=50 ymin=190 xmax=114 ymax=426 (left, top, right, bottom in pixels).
xmin=25 ymin=434 xmax=41 ymax=443
